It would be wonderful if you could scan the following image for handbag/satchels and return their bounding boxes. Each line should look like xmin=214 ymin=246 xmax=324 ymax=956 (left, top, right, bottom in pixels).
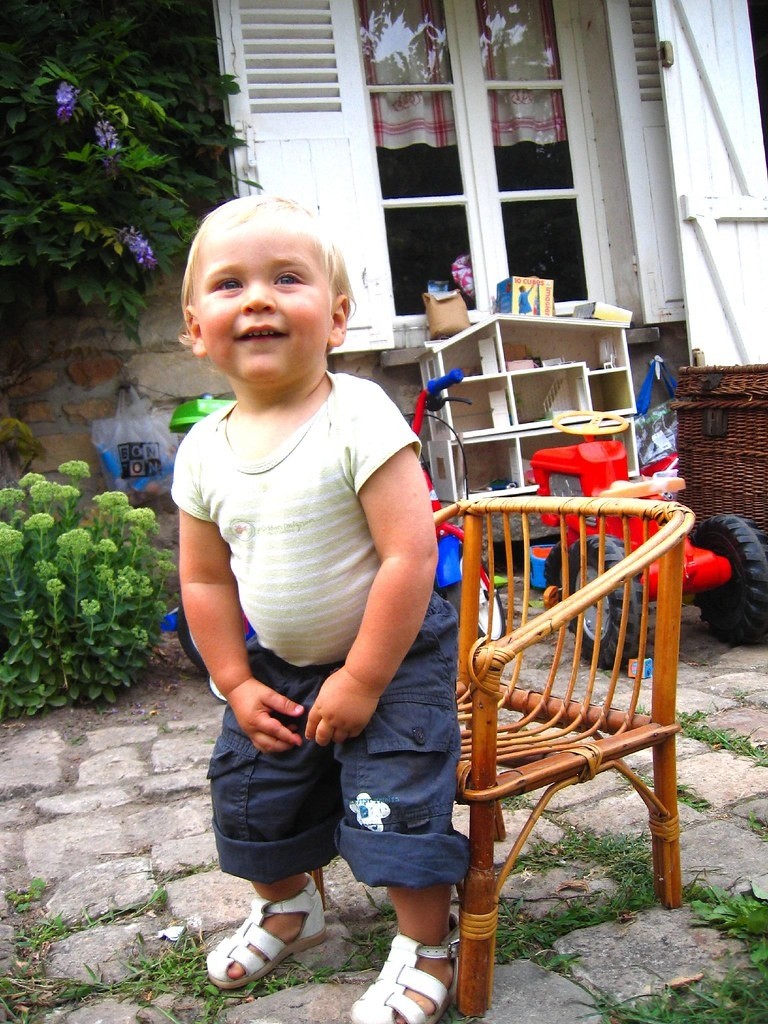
xmin=91 ymin=385 xmax=180 ymax=510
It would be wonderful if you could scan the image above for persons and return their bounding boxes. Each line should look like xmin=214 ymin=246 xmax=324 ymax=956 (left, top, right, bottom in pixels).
xmin=518 ymin=283 xmax=534 ymax=316
xmin=167 ymin=189 xmax=461 ymax=1024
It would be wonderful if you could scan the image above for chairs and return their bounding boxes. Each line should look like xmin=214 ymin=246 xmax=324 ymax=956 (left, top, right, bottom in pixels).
xmin=308 ymin=496 xmax=696 ymax=1018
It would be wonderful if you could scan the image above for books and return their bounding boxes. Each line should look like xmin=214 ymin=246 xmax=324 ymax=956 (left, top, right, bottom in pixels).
xmin=572 ymin=302 xmax=633 ymax=324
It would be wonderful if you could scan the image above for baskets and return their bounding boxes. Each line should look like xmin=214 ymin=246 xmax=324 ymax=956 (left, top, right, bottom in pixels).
xmin=667 ymin=364 xmax=768 ymax=542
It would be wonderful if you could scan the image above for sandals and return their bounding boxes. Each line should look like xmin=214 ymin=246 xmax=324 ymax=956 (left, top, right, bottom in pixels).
xmin=207 ymin=873 xmax=326 ymax=989
xmin=350 ymin=910 xmax=460 ymax=1024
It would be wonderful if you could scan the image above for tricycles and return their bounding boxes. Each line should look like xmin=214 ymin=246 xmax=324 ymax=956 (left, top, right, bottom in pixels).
xmin=162 ymin=366 xmax=508 ymax=700
xmin=525 ymin=409 xmax=767 ymax=672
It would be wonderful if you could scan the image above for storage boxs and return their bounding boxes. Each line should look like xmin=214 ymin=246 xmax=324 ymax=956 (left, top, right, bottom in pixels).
xmin=573 ymin=301 xmax=633 ymax=323
xmin=670 ymin=363 xmax=768 ymax=538
xmin=497 ymin=276 xmax=555 ymax=318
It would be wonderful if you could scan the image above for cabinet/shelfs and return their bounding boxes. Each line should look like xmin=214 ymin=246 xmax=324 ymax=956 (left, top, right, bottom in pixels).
xmin=426 ymin=417 xmax=641 ymax=504
xmin=414 ymin=311 xmax=638 ymax=441
